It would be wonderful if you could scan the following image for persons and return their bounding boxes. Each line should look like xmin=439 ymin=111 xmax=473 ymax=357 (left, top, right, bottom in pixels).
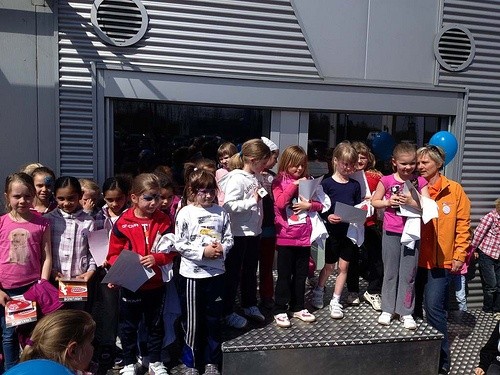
xmin=212 ymin=136 xmax=319 ymax=328
xmin=412 ymin=143 xmax=471 ymax=374
xmin=0 ymin=159 xmax=220 ymax=375
xmin=377 ymin=141 xmax=430 ymax=330
xmin=474 ymin=318 xmax=500 ymax=375
xmin=446 ymin=228 xmax=478 ymax=314
xmin=471 ymin=196 xmax=500 ymax=320
xmin=347 ymin=141 xmax=386 ymax=311
xmin=315 ymin=142 xmax=363 ymax=319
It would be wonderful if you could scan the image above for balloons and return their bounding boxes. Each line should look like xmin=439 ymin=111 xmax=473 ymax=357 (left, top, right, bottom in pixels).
xmin=429 ymin=131 xmax=459 ymax=168
xmin=373 ymin=132 xmax=394 ymax=165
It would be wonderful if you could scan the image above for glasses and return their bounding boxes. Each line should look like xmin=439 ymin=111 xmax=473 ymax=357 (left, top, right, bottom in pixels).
xmin=424 ymin=143 xmax=442 ymax=158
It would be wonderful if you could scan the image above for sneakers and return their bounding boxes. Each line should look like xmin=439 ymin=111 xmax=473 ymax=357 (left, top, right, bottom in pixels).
xmin=348 ymin=292 xmax=359 ymax=305
xmin=273 ymin=310 xmax=290 ymax=327
xmin=221 ymin=312 xmax=248 ymax=328
xmin=136 ymin=356 xmax=143 ymax=368
xmin=400 ymin=314 xmax=417 ymax=329
xmin=364 ymin=291 xmax=382 ymax=311
xmin=243 ymin=306 xmax=264 ymax=320
xmin=288 ymin=308 xmax=315 ymax=321
xmin=184 ymin=367 xmax=199 ymax=375
xmin=378 ymin=311 xmax=395 ymax=325
xmin=120 ymin=365 xmax=137 ymax=375
xmin=203 ymin=364 xmax=221 ymax=375
xmin=313 ymin=288 xmax=325 ymax=309
xmin=329 ymin=300 xmax=343 ymax=318
xmin=148 ymin=362 xmax=168 ymax=375
